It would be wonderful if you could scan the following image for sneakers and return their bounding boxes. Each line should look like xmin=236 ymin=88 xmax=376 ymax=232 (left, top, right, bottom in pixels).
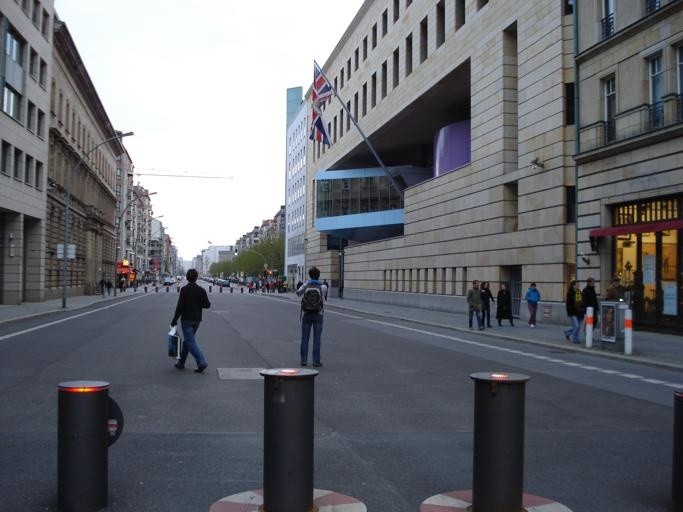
xmin=564 ymin=330 xmax=581 ymax=343
xmin=194 ymin=366 xmax=204 ymax=372
xmin=301 ymin=361 xmax=307 ymax=365
xmin=313 ymin=362 xmax=320 ymax=366
xmin=469 ymin=325 xmax=491 ymax=330
xmin=175 ymin=362 xmax=184 ymax=369
xmin=528 ymin=324 xmax=536 ymax=328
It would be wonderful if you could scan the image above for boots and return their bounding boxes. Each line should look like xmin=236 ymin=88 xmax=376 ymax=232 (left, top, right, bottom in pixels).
xmin=510 ymin=319 xmax=514 ymax=326
xmin=499 ymin=319 xmax=501 ymax=326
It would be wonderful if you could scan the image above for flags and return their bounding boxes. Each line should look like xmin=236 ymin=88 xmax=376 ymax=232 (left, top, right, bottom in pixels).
xmin=309 ymin=65 xmax=333 ymax=146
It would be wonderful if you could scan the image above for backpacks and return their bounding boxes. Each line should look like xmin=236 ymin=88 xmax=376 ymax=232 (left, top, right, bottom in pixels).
xmin=301 ymin=284 xmax=323 ymax=312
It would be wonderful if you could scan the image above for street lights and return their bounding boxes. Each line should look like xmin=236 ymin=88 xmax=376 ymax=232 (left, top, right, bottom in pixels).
xmin=59 ymin=127 xmax=135 ymax=311
xmin=115 ymin=190 xmax=176 ymax=272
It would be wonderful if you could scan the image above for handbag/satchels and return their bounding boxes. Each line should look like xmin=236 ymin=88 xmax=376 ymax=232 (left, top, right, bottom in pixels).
xmin=168 ymin=334 xmax=181 ymax=359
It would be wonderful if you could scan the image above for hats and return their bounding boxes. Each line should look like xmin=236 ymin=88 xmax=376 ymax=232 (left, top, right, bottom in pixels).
xmin=612 ymin=276 xmax=620 ymax=281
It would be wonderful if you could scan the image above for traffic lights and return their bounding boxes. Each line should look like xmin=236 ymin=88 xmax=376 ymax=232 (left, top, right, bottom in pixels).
xmin=265 ymin=270 xmax=272 ymax=274
xmin=234 ymin=247 xmax=237 ymax=255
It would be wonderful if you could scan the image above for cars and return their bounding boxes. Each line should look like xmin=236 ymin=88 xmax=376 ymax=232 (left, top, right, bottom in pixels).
xmin=163 ymin=276 xmax=182 ymax=286
xmin=198 ymin=275 xmax=229 ymax=288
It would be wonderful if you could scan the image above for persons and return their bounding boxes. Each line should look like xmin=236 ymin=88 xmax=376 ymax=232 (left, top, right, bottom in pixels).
xmin=480 ymin=281 xmax=496 ymax=328
xmin=580 ymin=277 xmax=600 ymax=333
xmin=296 ymin=266 xmax=328 ymax=367
xmin=526 ymin=282 xmax=541 ymax=328
xmin=297 ymin=280 xmax=303 ymax=296
xmin=170 ymin=269 xmax=211 ymax=373
xmin=564 ymin=280 xmax=582 ymax=345
xmin=245 ymin=269 xmax=288 ymax=293
xmin=496 ymin=284 xmax=515 ymax=326
xmin=603 ymin=307 xmax=613 ymax=340
xmin=100 ymin=277 xmax=107 ymax=294
xmin=603 ymin=277 xmax=621 ymax=301
xmin=466 ymin=280 xmax=485 ymax=330
xmin=106 ymin=278 xmax=114 ymax=295
xmin=121 ymin=275 xmax=126 ymax=291
